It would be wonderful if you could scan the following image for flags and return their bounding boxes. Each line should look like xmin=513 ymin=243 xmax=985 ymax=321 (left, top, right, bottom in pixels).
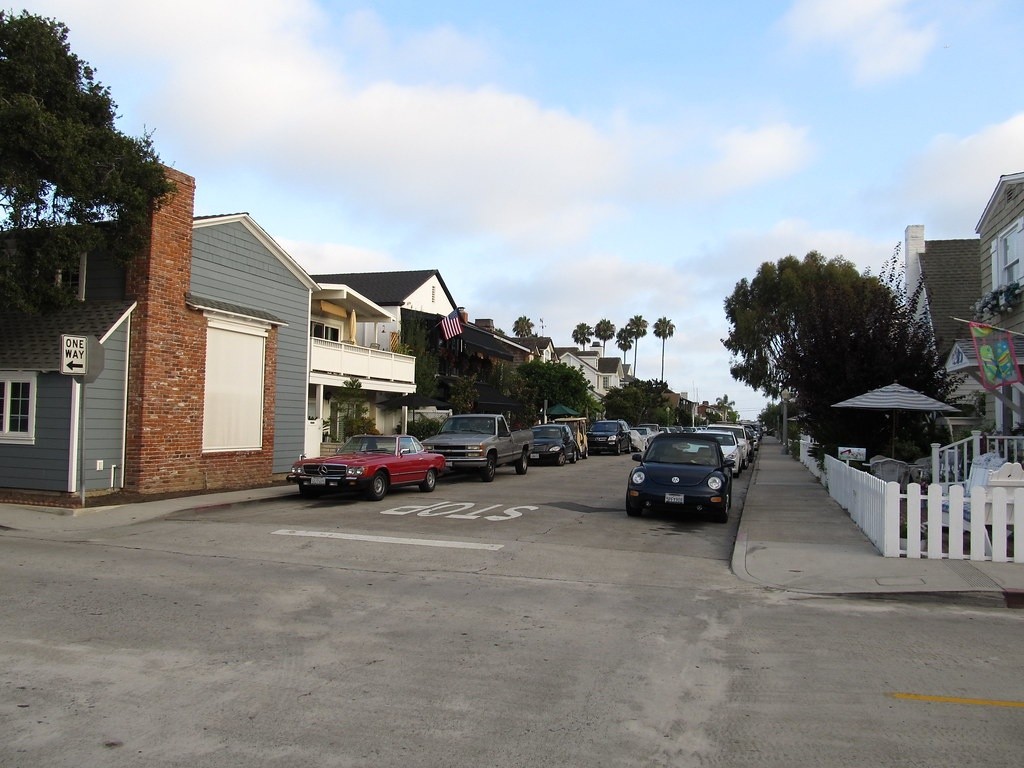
xmin=969 ymin=321 xmax=1023 ymax=390
xmin=442 ymin=309 xmax=464 ymax=340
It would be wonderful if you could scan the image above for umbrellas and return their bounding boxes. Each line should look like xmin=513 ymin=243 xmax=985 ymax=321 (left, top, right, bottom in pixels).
xmin=537 ymin=404 xmax=580 ymax=416
xmin=830 ymin=380 xmax=962 ymax=460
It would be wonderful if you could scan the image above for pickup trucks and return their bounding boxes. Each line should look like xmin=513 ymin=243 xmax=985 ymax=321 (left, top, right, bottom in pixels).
xmin=420 ymin=414 xmax=535 ymax=482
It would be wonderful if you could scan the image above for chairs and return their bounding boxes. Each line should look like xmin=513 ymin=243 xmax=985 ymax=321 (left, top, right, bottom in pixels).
xmin=861 ymin=450 xmax=961 ymax=491
xmin=927 ymin=452 xmax=1024 ymax=557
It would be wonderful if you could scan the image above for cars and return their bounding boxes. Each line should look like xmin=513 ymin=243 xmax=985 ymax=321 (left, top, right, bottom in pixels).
xmin=286 ymin=434 xmax=447 ymax=501
xmin=625 ymin=433 xmax=735 ymax=524
xmin=632 ymin=422 xmax=710 ymax=450
xmin=694 ymin=430 xmax=744 ymax=479
xmin=586 ymin=419 xmax=632 ymax=455
xmin=556 ymin=417 xmax=589 ymax=459
xmin=707 ymin=424 xmax=751 ymax=469
xmin=528 ymin=424 xmax=579 ymax=466
xmin=740 ymin=423 xmax=765 ymax=462
